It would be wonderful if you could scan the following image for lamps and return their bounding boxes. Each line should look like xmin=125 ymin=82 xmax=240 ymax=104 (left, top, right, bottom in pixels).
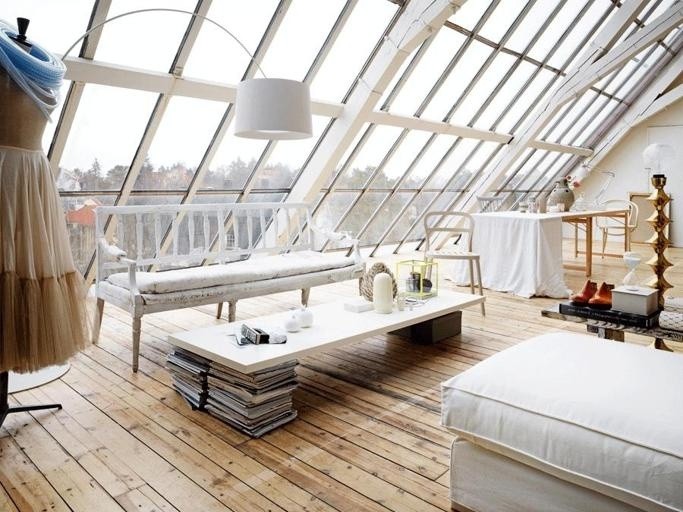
xmin=643 ymin=143 xmax=676 ymax=312
xmin=62 ymin=9 xmax=313 ymax=139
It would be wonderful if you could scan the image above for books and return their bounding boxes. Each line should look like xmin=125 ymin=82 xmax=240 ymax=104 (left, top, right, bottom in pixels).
xmin=163 ymin=343 xmax=301 ymax=439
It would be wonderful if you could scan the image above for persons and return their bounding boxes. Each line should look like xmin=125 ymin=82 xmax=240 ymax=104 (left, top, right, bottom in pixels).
xmin=1 ymin=36 xmax=94 ymax=374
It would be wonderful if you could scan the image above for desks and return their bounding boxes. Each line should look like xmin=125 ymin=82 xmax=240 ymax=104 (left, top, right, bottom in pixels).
xmin=167 ymin=288 xmax=486 ymax=375
xmin=466 ymin=209 xmax=631 ymax=299
xmin=541 ymin=304 xmax=683 ymax=352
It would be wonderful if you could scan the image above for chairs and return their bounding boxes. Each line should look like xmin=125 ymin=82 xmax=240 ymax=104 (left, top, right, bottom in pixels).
xmin=476 ymin=195 xmax=505 ymax=213
xmin=594 ymin=198 xmax=639 ymax=259
xmin=422 ymin=211 xmax=486 ymax=315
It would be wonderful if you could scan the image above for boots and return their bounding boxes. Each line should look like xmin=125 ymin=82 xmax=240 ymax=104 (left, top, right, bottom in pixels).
xmin=589 ymin=283 xmax=615 ymax=306
xmin=572 ymin=280 xmax=597 ymax=303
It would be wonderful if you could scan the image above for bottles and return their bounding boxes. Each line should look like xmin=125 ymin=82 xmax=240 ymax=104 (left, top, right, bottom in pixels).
xmin=574 ymin=193 xmax=588 ymax=213
xmin=528 ymin=197 xmax=536 ymax=214
xmin=540 ymin=193 xmax=547 ymax=213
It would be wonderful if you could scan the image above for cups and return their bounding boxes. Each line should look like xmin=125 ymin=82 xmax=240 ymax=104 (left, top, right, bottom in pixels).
xmin=519 ymin=201 xmax=528 ymax=213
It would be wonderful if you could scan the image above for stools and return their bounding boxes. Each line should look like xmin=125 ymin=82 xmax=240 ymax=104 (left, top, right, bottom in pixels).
xmin=440 ymin=330 xmax=683 ymax=512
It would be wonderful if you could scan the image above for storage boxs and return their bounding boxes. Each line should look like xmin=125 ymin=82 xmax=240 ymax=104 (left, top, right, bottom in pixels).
xmin=610 ymin=285 xmax=660 ymax=317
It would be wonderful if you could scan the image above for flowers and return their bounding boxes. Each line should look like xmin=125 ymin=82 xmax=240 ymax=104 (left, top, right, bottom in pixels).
xmin=566 ymin=175 xmax=582 ymax=189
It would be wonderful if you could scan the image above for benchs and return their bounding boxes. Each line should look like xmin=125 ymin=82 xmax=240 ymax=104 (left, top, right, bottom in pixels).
xmin=92 ymin=200 xmax=366 ymax=373
